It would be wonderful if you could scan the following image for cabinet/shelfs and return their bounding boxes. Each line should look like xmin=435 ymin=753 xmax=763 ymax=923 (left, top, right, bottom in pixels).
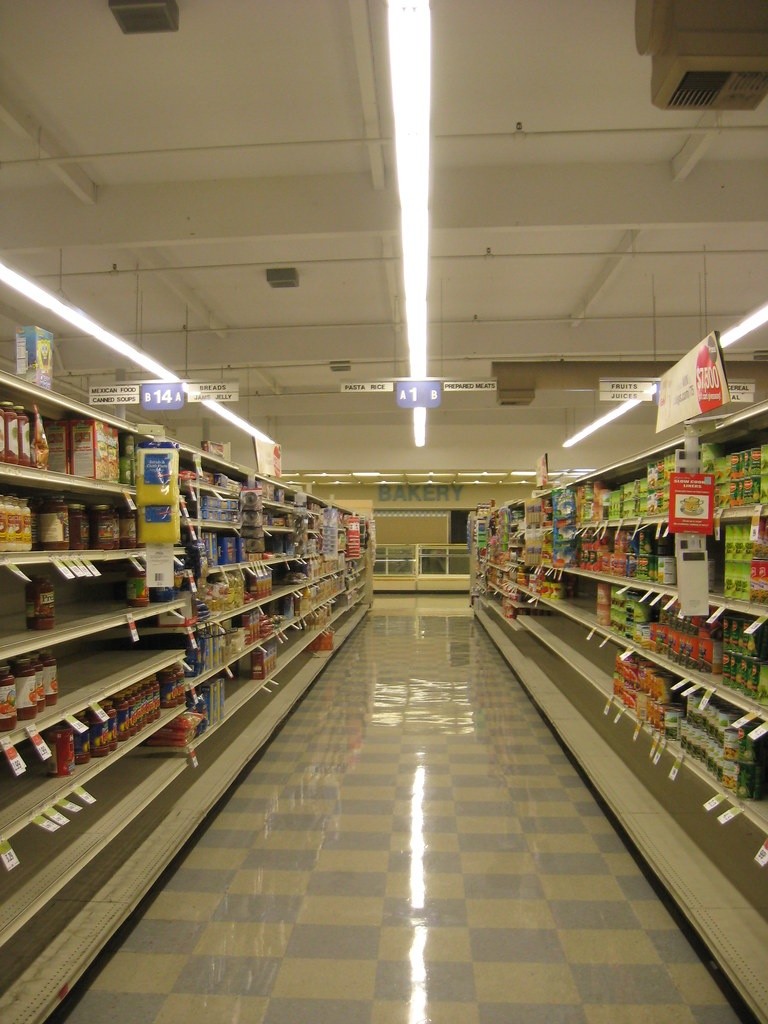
xmin=468 ymin=397 xmax=768 ymax=1024
xmin=0 ymin=397 xmax=183 ymax=1024
xmin=417 ymin=544 xmax=469 ymax=593
xmin=373 ymin=544 xmax=418 ymax=593
xmin=175 ymin=443 xmax=372 ymax=763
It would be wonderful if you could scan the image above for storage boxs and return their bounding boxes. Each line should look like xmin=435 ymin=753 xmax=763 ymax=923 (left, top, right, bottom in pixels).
xmin=16 ymin=325 xmax=53 ymax=391
xmin=41 ymin=418 xmax=123 ymax=483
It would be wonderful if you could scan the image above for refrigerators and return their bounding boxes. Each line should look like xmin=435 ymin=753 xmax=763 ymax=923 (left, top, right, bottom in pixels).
xmin=374 ymin=544 xmax=417 ymax=590
xmin=415 ymin=543 xmax=472 ymax=591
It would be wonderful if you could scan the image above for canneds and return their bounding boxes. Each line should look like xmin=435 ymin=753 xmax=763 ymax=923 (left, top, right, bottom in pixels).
xmin=0 ymin=491 xmax=188 ymax=780
xmin=542 ymin=447 xmax=768 ymax=800
